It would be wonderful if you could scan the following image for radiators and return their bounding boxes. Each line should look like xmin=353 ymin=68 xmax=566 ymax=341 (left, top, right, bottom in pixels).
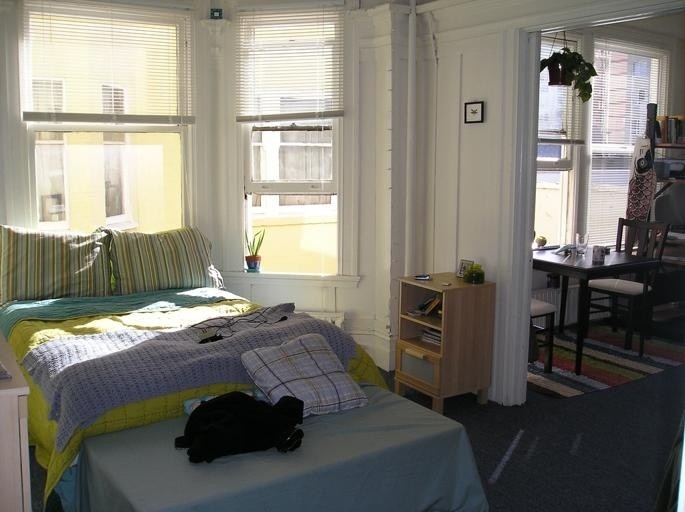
xmin=530 ymin=282 xmax=612 ymax=331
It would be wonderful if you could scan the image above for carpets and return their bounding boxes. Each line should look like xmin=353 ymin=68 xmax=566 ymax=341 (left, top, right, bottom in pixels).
xmin=525 ymin=300 xmax=685 ymax=397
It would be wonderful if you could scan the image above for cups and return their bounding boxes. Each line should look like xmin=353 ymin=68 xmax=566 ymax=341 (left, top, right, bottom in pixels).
xmin=592 ymin=245 xmax=606 ymax=265
xmin=575 ymin=232 xmax=590 ymax=255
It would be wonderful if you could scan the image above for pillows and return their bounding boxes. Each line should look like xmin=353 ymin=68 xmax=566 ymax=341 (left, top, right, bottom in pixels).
xmin=96 ymin=223 xmax=219 ymax=301
xmin=237 ymin=329 xmax=374 ymax=423
xmin=0 ymin=223 xmax=119 ymax=302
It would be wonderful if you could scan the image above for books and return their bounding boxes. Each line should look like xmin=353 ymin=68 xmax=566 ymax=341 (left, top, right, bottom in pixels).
xmin=419 ymin=327 xmax=442 ymax=347
xmin=656 ymin=116 xmax=685 ymax=146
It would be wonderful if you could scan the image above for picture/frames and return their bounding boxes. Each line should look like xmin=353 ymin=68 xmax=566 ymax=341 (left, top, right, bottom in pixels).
xmin=463 ymin=98 xmax=486 ymax=125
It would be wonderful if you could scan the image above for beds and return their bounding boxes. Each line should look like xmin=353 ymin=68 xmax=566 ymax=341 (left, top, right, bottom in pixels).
xmin=0 ymin=220 xmax=494 ymax=511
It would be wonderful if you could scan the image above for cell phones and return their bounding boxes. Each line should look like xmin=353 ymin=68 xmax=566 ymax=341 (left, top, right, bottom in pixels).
xmin=414 ymin=274 xmax=430 ymax=280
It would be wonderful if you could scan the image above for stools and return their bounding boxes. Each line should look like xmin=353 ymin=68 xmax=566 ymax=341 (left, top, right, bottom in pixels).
xmin=529 ymin=298 xmax=558 ymax=376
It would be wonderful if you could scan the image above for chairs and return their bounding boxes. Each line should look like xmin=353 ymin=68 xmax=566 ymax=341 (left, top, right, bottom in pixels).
xmin=575 ymin=217 xmax=673 ymax=359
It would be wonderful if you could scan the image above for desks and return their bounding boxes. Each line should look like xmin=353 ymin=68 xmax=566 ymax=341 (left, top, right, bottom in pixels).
xmin=533 ymin=239 xmax=665 ymax=379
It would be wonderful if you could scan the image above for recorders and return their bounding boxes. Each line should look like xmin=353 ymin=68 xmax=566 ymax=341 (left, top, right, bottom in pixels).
xmin=653 ymin=158 xmax=685 ymax=182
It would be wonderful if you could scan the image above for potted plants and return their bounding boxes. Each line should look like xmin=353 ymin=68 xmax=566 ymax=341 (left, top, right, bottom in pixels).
xmin=541 ymin=44 xmax=601 ymax=105
xmin=240 ymin=228 xmax=268 ymax=275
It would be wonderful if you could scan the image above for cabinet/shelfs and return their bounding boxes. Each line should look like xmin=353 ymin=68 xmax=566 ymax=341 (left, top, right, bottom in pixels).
xmin=644 ymin=102 xmax=685 ymax=257
xmin=389 ymin=270 xmax=498 ymax=419
xmin=0 ymin=324 xmax=37 ymax=511
